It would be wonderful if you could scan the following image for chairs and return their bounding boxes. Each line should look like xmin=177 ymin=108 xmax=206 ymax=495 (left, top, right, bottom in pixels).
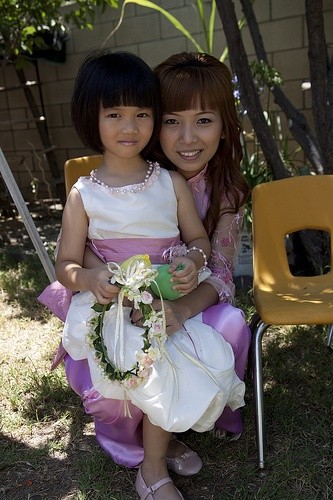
xmin=248 ymin=175 xmax=333 ymax=478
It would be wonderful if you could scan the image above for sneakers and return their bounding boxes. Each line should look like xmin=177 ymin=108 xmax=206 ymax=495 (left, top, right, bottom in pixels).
xmin=165 ymin=434 xmax=202 ymax=476
xmin=134 ymin=463 xmax=185 ymax=500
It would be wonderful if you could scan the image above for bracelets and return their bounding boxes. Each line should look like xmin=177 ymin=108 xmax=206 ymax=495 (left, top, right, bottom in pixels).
xmin=186 ymin=247 xmax=208 ymax=275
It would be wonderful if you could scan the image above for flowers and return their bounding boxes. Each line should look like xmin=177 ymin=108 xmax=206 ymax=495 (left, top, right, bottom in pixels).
xmin=88 ymin=281 xmax=163 ymax=389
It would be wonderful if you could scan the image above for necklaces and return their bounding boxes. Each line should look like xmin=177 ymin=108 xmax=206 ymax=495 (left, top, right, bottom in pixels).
xmin=90 ymin=160 xmax=153 ymax=193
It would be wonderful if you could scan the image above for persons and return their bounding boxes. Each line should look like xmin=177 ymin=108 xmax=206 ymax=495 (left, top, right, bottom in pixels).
xmin=55 ymin=50 xmax=246 ymax=500
xmin=38 ymin=51 xmax=254 ymax=469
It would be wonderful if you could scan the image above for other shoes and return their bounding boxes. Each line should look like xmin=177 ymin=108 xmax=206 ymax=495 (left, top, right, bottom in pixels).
xmin=208 ymin=424 xmax=242 ymax=442
